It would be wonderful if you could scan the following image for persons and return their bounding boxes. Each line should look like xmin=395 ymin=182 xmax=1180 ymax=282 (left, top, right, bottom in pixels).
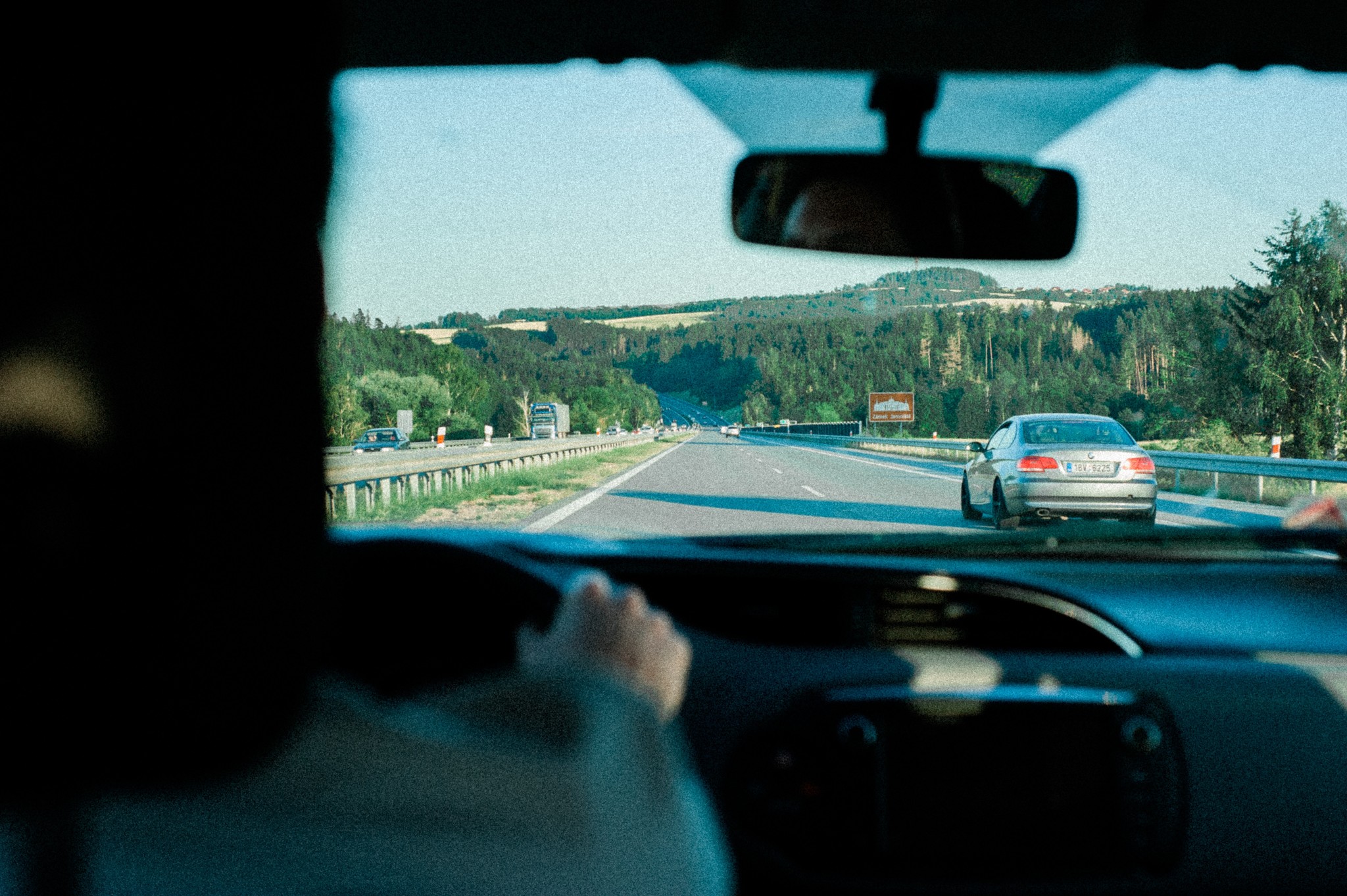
xmin=96 ymin=571 xmax=738 ymax=896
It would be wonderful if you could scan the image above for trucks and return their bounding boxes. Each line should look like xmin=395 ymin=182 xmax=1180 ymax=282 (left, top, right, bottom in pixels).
xmin=529 ymin=403 xmax=570 ymax=440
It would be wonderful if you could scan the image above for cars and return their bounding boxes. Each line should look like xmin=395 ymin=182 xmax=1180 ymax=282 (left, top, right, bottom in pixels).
xmin=642 ymin=426 xmax=651 ymax=430
xmin=352 ymin=427 xmax=410 ymax=452
xmin=610 ymin=428 xmax=617 ymax=435
xmin=961 ymin=413 xmax=1159 ymax=530
xmin=726 ymin=425 xmax=740 ymax=438
xmin=719 ymin=425 xmax=729 ymax=435
